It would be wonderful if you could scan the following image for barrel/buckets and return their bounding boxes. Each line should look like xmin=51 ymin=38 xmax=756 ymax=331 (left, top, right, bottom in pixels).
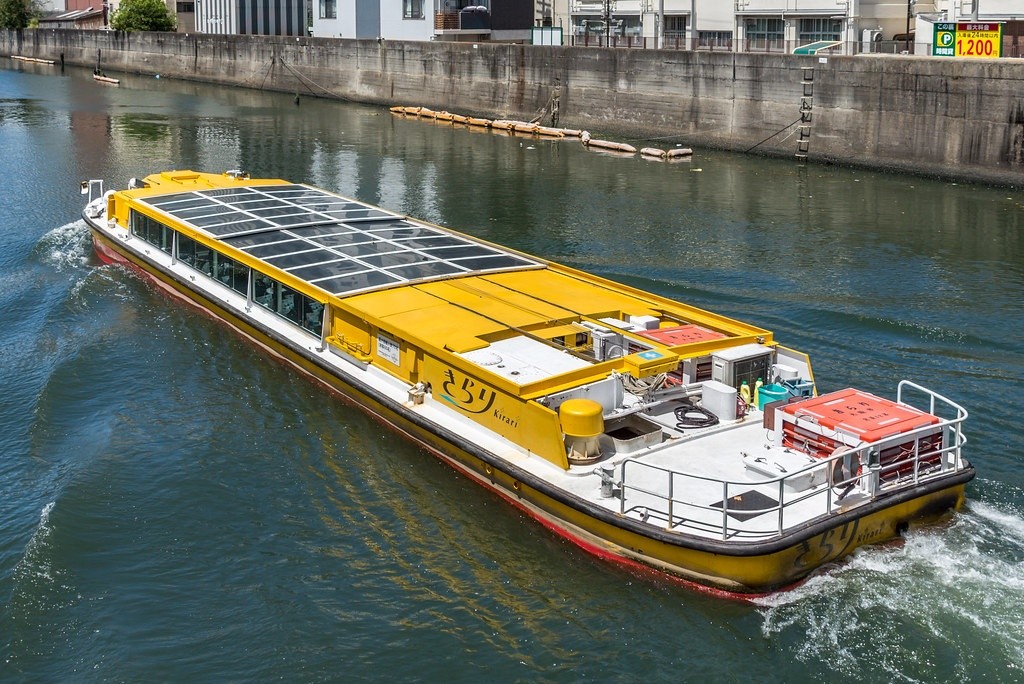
xmin=754 ymin=377 xmax=763 ymax=406
xmin=757 ymin=384 xmax=789 ymax=412
xmin=740 ymin=381 xmax=751 ymax=404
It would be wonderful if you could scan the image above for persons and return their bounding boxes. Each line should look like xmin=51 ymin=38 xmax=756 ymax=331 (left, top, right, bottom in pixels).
xmin=584 ymin=20 xmax=589 ymax=46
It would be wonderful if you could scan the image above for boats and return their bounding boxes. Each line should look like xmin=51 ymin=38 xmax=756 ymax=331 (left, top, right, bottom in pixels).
xmin=80 ymin=169 xmax=980 ymax=601
